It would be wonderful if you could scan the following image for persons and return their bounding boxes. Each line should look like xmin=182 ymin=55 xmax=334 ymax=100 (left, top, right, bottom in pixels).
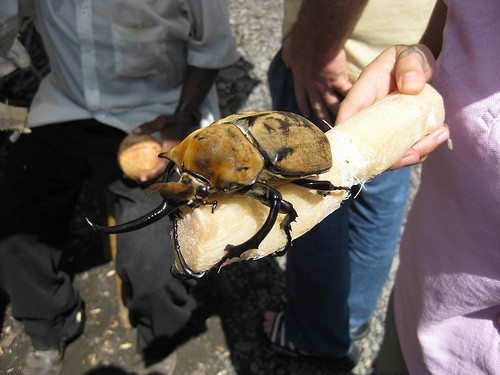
xmin=260 ymin=1 xmax=451 ymax=365
xmin=1 ymin=1 xmax=243 ymax=375
xmin=332 ymin=1 xmax=500 ymax=375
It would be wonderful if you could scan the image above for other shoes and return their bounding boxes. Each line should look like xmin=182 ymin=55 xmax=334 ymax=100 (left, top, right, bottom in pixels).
xmin=132 ymin=349 xmax=177 ymax=375
xmin=22 ymin=344 xmax=65 ymax=375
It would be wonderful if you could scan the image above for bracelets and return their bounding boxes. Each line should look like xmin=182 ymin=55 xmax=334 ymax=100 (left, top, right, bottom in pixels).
xmin=174 ymin=98 xmax=202 ymax=126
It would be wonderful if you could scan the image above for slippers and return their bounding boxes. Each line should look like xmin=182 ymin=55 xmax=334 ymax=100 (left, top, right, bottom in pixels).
xmin=348 ymin=321 xmax=372 ymax=340
xmin=257 ymin=305 xmax=361 ymax=373
